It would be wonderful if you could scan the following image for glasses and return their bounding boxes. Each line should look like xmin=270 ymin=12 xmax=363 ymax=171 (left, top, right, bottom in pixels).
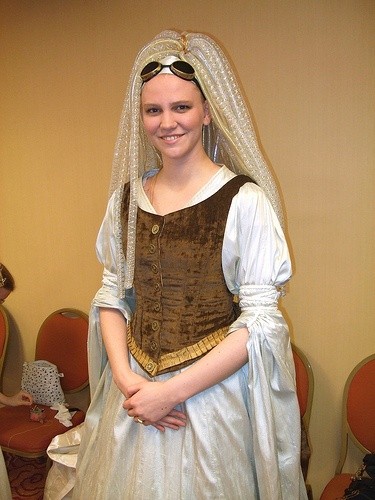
xmin=139 ymin=60 xmax=207 ymax=100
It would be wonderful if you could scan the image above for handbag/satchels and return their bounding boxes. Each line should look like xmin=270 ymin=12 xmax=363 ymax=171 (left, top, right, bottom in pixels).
xmin=21 ymin=360 xmax=67 ymax=408
xmin=341 ymin=452 xmax=375 ymax=500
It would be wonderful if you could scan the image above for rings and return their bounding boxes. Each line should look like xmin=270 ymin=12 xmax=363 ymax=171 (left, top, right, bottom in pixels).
xmin=137 ymin=417 xmax=144 ymax=425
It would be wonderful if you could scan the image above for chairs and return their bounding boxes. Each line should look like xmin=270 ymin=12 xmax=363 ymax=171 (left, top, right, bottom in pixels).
xmin=319 ymin=354 xmax=375 ymax=500
xmin=0 ymin=308 xmax=89 ymax=479
xmin=290 ymin=341 xmax=314 ymax=500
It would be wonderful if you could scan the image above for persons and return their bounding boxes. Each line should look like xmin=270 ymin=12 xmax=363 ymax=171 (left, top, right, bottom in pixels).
xmin=47 ymin=29 xmax=310 ymax=500
xmin=0 ymin=263 xmax=35 ymax=500
